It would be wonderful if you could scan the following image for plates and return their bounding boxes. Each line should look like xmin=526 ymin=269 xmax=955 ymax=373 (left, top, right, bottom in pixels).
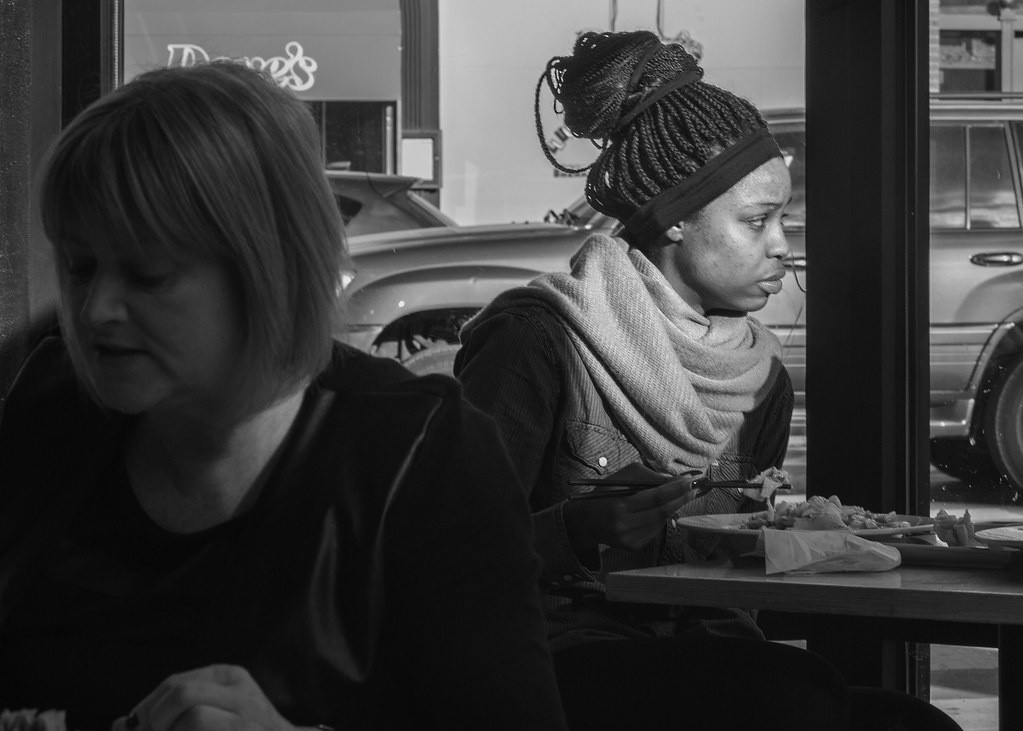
xmin=675 ymin=510 xmax=939 ymax=548
xmin=974 ymin=526 xmax=1023 ymax=553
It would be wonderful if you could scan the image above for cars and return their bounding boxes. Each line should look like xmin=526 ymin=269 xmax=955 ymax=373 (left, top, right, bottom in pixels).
xmin=326 ymin=170 xmax=457 ymax=235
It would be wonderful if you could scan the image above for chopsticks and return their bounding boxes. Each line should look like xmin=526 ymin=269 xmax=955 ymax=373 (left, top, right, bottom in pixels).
xmin=565 ymin=476 xmax=792 ymax=502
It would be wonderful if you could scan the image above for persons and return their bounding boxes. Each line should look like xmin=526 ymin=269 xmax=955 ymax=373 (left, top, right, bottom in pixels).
xmin=453 ymin=31 xmax=963 ymax=731
xmin=0 ymin=58 xmax=569 ymax=731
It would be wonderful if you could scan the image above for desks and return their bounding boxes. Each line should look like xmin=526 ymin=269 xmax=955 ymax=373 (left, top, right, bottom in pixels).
xmin=602 ymin=559 xmax=1023 ymax=731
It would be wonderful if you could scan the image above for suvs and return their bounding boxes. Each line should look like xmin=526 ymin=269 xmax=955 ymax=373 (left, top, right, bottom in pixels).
xmin=331 ymin=93 xmax=1023 ymax=506
